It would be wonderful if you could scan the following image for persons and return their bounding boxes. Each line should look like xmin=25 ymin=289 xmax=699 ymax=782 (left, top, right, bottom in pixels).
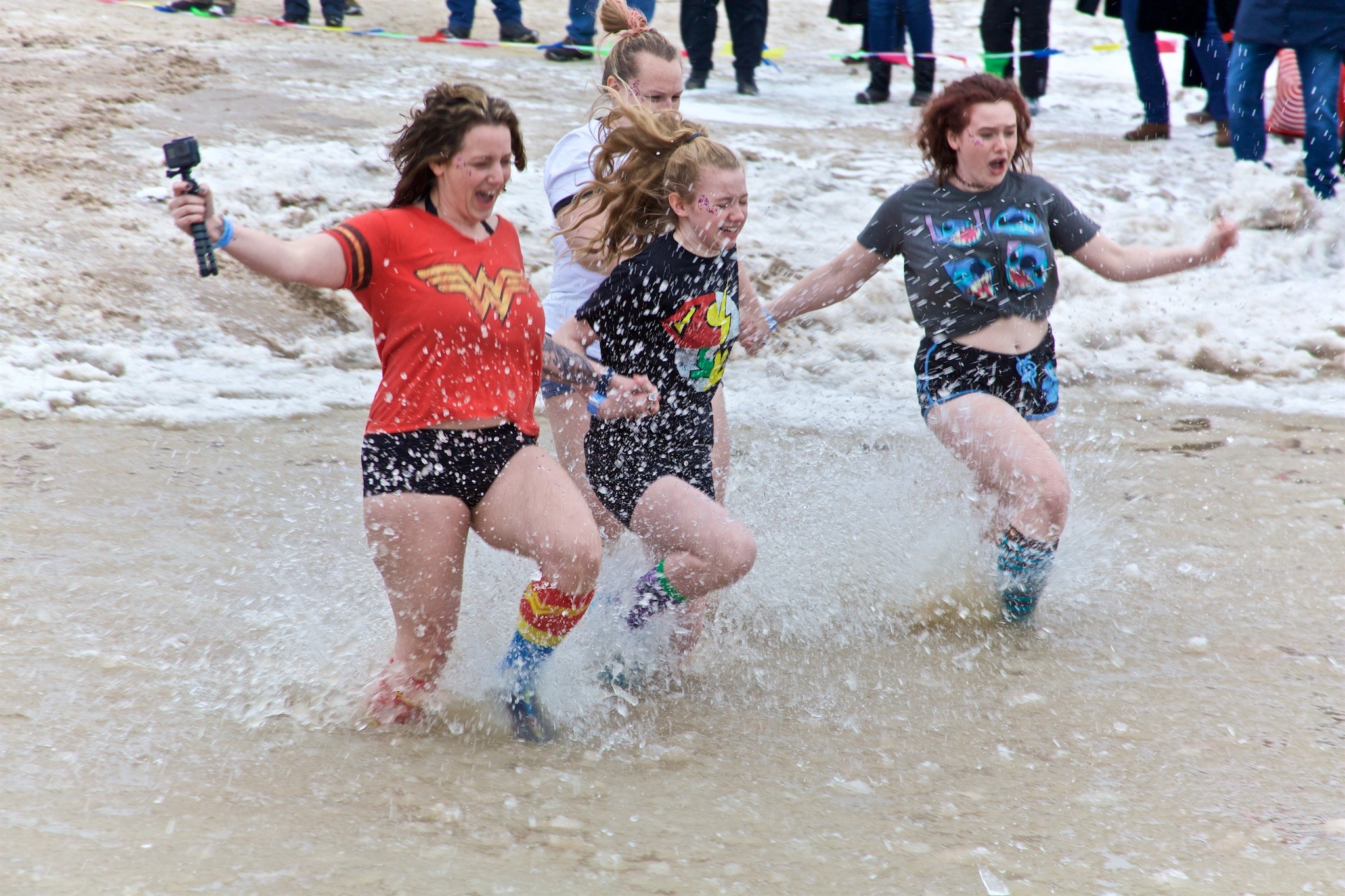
xmin=171 ymin=83 xmax=646 ymax=739
xmin=679 ymin=0 xmax=769 ymax=94
xmin=545 ymin=0 xmax=656 ymax=61
xmin=279 ymin=0 xmax=362 ymax=28
xmin=857 ymin=0 xmax=935 ymax=107
xmin=742 ymin=74 xmax=1237 ymax=620
xmin=551 ymin=81 xmax=770 ymax=631
xmin=1123 ymin=0 xmax=1345 ymax=201
xmin=440 ymin=0 xmax=540 ymax=42
xmin=541 ymin=0 xmax=733 ymax=543
xmin=980 ymin=0 xmax=1053 ymax=116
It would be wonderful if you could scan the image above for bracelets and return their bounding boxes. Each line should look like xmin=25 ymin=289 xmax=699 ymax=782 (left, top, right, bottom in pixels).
xmin=596 ymin=366 xmax=614 ymax=395
xmin=761 ymin=308 xmax=777 ymax=332
xmin=587 ymin=393 xmax=607 ymax=419
xmin=208 ymin=216 xmax=234 ymax=249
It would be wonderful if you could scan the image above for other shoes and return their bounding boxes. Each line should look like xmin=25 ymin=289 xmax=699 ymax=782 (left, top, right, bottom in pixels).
xmin=545 ymin=37 xmax=593 ymax=61
xmin=1186 ymin=110 xmax=1215 ymax=125
xmin=1026 ymin=97 xmax=1039 ymax=117
xmin=210 ymin=3 xmax=233 ymax=15
xmin=165 ymin=0 xmax=209 ymax=11
xmin=506 ymin=693 xmax=557 ymax=747
xmin=437 ymin=27 xmax=457 ymax=39
xmin=1125 ymin=122 xmax=1171 ymax=141
xmin=684 ymin=78 xmax=705 ymax=90
xmin=325 ymin=15 xmax=343 ymax=27
xmin=738 ymin=78 xmax=758 ymax=95
xmin=856 ymin=91 xmax=881 ymax=104
xmin=1215 ymin=120 xmax=1232 ymax=147
xmin=342 ymin=1 xmax=362 ymax=14
xmin=908 ymin=90 xmax=930 ymax=107
xmin=501 ymin=30 xmax=539 ymax=43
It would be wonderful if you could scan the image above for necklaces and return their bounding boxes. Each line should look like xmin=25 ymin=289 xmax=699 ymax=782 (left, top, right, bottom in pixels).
xmin=954 ymin=171 xmax=985 ymax=189
